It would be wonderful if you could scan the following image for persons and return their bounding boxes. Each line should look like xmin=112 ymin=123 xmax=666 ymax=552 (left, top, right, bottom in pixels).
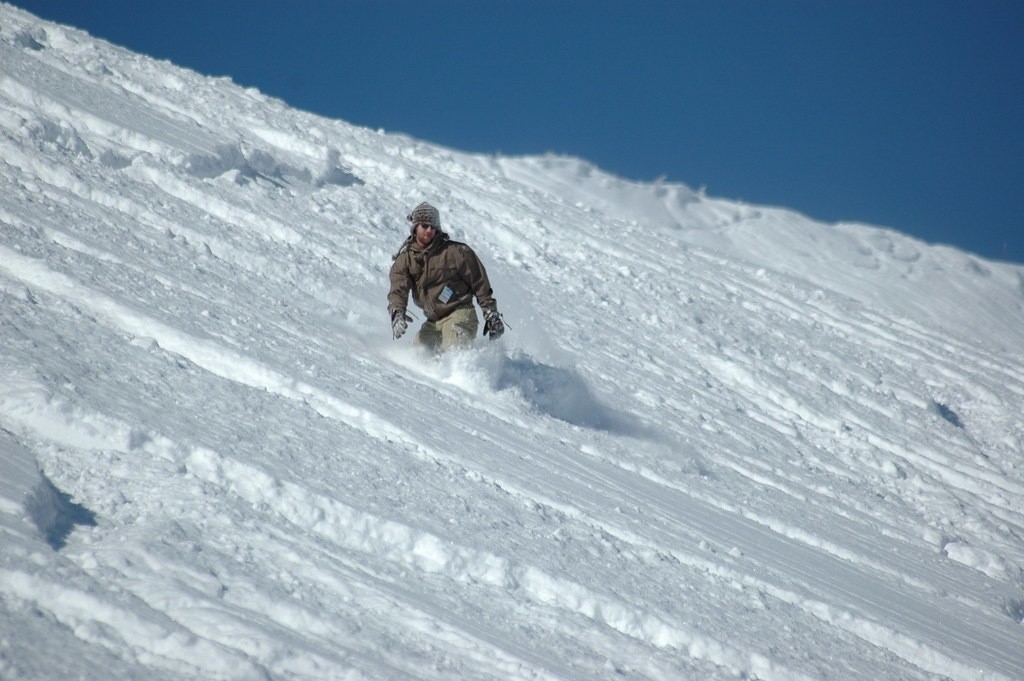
xmin=386 ymin=202 xmax=504 ymax=350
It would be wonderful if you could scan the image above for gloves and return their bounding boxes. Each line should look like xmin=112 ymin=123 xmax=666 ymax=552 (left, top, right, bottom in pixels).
xmin=392 ymin=312 xmax=413 ymax=339
xmin=483 ymin=311 xmax=505 ymax=342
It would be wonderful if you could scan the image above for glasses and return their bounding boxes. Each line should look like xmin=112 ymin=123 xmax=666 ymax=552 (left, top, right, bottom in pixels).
xmin=422 ymin=224 xmax=435 ymax=229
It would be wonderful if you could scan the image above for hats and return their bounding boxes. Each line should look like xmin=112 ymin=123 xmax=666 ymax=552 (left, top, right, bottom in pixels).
xmin=392 ymin=202 xmax=442 ymax=261
